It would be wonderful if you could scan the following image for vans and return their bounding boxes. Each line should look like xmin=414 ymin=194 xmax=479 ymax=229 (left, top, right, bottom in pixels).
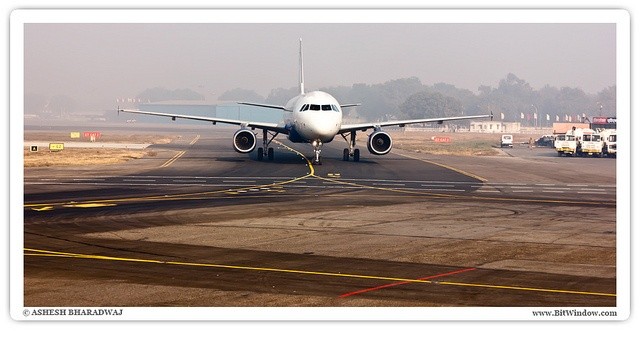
xmin=501 ymin=135 xmax=513 ymax=148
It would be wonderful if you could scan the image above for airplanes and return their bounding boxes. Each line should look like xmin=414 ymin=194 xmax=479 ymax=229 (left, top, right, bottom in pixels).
xmin=115 ymin=37 xmax=490 ymax=164
